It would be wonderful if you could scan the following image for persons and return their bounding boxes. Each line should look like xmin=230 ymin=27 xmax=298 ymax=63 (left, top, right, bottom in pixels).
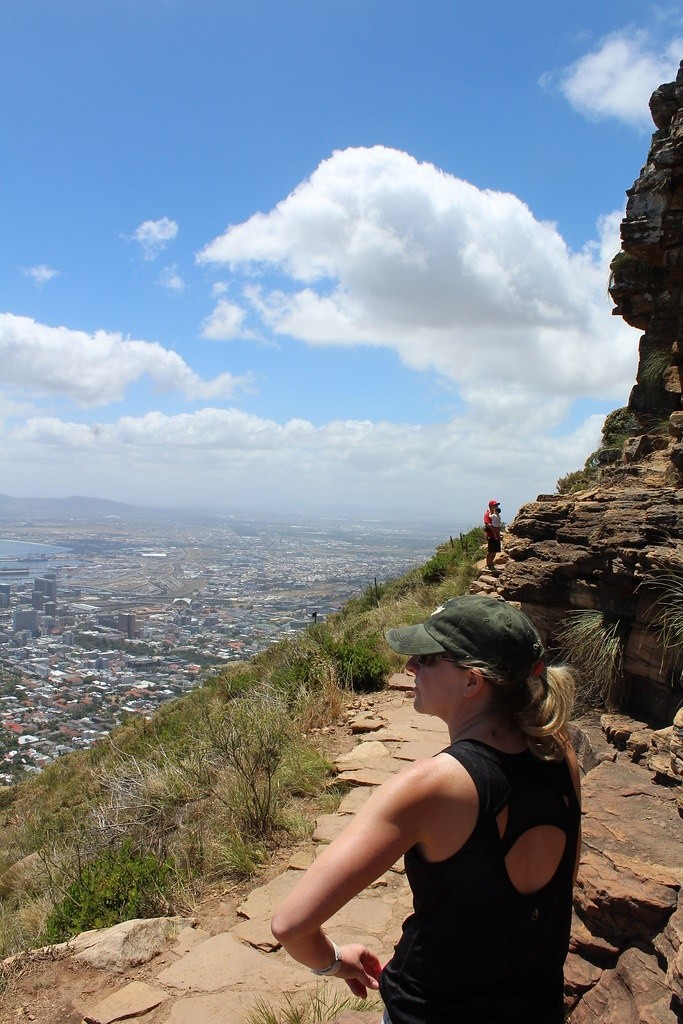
xmin=267 ymin=597 xmax=581 ymax=1024
xmin=483 ymin=501 xmax=501 ymax=569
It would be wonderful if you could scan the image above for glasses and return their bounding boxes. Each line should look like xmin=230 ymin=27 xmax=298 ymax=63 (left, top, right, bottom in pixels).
xmin=417 ymin=653 xmax=459 ymax=667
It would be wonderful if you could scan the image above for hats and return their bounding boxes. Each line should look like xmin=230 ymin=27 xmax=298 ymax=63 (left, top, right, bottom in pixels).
xmin=384 ymin=594 xmax=547 ymax=687
xmin=488 ymin=501 xmax=500 ymax=507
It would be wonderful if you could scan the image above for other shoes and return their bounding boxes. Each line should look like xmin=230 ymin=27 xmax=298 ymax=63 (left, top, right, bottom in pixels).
xmin=486 ymin=563 xmax=497 ymax=570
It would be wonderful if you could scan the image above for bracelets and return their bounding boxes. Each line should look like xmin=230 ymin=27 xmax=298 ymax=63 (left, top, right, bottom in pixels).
xmin=311 ymin=937 xmax=344 ymax=977
